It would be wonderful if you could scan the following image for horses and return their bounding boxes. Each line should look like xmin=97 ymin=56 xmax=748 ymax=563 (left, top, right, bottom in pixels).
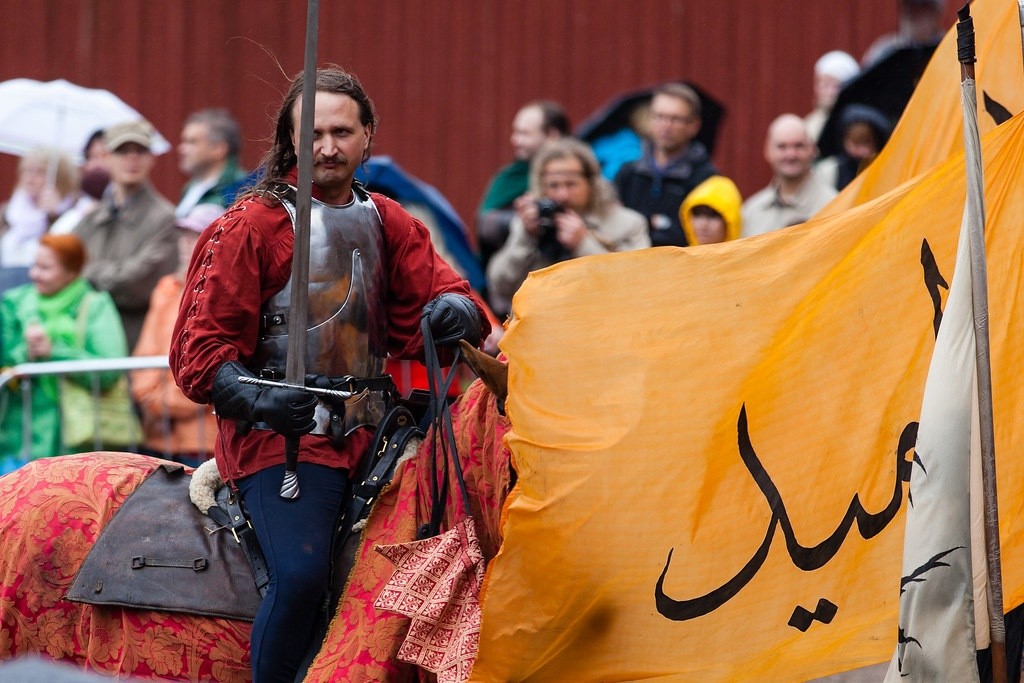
xmin=0 ymin=337 xmax=508 ymax=682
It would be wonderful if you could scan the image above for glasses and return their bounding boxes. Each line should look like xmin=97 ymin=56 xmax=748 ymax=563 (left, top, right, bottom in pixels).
xmin=649 ymin=112 xmax=695 ymax=125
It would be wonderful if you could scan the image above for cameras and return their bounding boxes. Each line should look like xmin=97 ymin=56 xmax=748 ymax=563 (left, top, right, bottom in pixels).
xmin=535 ymin=199 xmax=565 ymax=219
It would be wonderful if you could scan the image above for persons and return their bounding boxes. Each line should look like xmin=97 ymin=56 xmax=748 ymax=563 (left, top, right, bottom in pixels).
xmin=473 ymin=81 xmax=741 ymax=327
xmin=1 ymin=107 xmax=254 ymax=476
xmin=168 ymin=66 xmax=491 ymax=683
xmin=743 ymin=0 xmax=951 ymax=254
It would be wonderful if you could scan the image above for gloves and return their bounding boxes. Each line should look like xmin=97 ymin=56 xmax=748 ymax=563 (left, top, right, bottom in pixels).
xmin=421 ymin=294 xmax=481 ymax=349
xmin=211 ymin=360 xmax=318 ymax=438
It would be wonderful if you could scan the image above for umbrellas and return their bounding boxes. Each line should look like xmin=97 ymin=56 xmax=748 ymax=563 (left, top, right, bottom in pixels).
xmin=0 ymin=78 xmax=172 ymax=211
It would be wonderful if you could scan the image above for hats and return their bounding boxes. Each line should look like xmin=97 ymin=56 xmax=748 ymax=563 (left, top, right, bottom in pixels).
xmin=104 ymin=121 xmax=154 ymax=154
xmin=816 ymin=51 xmax=860 ymax=83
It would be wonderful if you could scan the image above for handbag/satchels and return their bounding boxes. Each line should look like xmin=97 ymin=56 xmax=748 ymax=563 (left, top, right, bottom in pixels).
xmin=57 ymin=294 xmax=143 ymax=448
